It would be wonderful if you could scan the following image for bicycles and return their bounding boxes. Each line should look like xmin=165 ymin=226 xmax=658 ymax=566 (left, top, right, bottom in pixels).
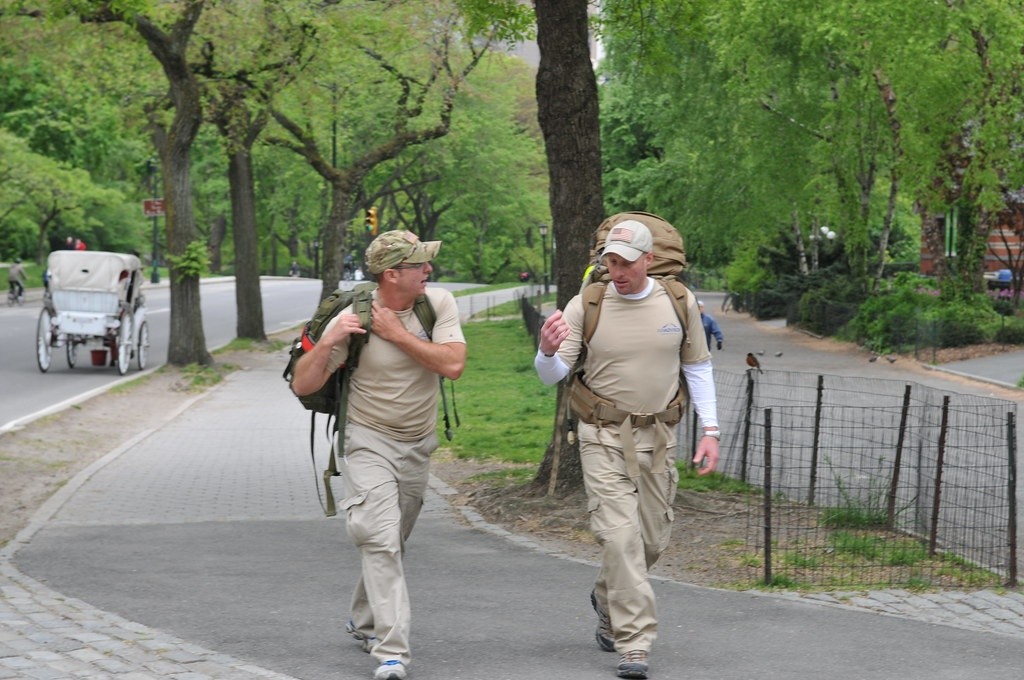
xmin=8 ymin=282 xmax=22 ymax=306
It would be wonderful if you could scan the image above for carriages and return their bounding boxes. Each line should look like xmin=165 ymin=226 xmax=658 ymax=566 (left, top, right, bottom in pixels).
xmin=35 ymin=249 xmax=150 ymax=376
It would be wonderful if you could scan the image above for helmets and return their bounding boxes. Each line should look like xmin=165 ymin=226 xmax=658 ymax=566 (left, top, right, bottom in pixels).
xmin=14 ymin=258 xmax=21 ymax=263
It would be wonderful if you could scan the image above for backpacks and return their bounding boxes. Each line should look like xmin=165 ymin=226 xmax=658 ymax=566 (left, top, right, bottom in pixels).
xmin=282 ymin=282 xmax=462 ymax=517
xmin=562 ymin=210 xmax=692 ymax=496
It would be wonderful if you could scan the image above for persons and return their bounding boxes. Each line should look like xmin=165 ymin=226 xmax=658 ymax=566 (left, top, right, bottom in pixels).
xmin=6 ymin=257 xmax=29 ymax=303
xmin=288 ymin=261 xmax=301 ymax=277
xmin=293 ymin=230 xmax=466 ymax=680
xmin=534 ymin=219 xmax=720 ymax=680
xmin=343 ymin=255 xmax=365 ymax=280
xmin=696 ymin=301 xmax=724 ymax=352
xmin=42 ymin=237 xmax=86 ymax=300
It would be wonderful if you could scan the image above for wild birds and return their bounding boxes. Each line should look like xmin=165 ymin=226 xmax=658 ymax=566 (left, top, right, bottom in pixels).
xmin=775 ymin=351 xmax=782 ymax=356
xmin=886 ymin=356 xmax=897 ymax=362
xmin=747 ymin=353 xmax=763 ymax=374
xmin=757 ymin=350 xmax=765 ymax=354
xmin=869 ymin=355 xmax=879 ymax=362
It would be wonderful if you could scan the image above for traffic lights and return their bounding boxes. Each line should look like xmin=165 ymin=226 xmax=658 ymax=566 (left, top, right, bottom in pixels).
xmin=366 ymin=206 xmax=378 ymax=236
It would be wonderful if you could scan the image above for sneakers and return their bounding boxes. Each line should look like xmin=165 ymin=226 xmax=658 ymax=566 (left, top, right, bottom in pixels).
xmin=616 ymin=649 xmax=648 ymax=679
xmin=346 ymin=621 xmax=380 ymax=655
xmin=373 ymin=660 xmax=406 ymax=680
xmin=591 ymin=588 xmax=615 ymax=652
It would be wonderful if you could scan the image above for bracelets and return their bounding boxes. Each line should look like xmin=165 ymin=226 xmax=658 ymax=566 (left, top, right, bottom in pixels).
xmin=703 ymin=430 xmax=720 ymax=440
xmin=538 ymin=347 xmax=554 ymax=357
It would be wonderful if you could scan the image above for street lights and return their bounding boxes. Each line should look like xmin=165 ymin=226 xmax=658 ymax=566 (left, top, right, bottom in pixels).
xmin=538 ymin=226 xmax=550 ymax=295
xmin=147 ymin=160 xmax=164 ymax=283
xmin=313 ymin=79 xmax=385 ymax=169
xmin=314 ymin=240 xmax=319 ymax=279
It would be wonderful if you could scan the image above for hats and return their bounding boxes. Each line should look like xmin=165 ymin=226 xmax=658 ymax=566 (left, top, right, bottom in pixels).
xmin=599 ymin=220 xmax=654 ymax=263
xmin=365 ymin=229 xmax=443 ymax=275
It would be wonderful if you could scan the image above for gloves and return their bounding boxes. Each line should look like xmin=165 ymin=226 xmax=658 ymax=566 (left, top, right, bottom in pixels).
xmin=717 ymin=342 xmax=720 ymax=349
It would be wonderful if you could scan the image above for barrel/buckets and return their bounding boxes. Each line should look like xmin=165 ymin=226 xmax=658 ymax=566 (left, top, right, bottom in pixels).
xmin=90 ymin=340 xmax=107 ymax=366
